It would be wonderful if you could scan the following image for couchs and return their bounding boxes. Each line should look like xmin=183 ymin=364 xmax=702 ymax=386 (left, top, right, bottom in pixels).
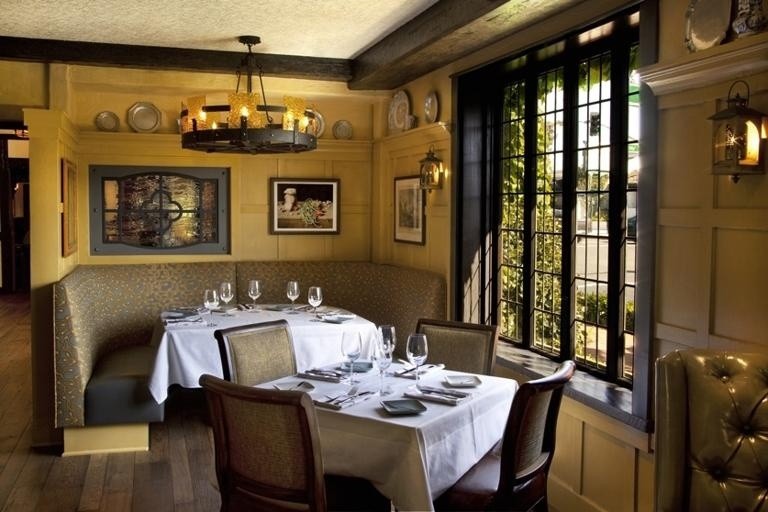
xmin=52 ymin=260 xmax=448 ymax=429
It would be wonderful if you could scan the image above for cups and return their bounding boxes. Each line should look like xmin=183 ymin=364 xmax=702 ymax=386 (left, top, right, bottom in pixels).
xmin=203 ymin=289 xmax=219 ymax=328
xmin=286 ymin=280 xmax=300 ymax=315
xmin=340 ymin=329 xmax=363 ymax=386
xmin=377 ymin=324 xmax=397 ymax=378
xmin=368 ymin=333 xmax=393 ymax=398
xmin=219 ymin=282 xmax=234 ymax=318
xmin=247 ymin=279 xmax=262 ymax=313
xmin=308 ymin=286 xmax=324 ymax=319
xmin=406 ymin=333 xmax=429 ymax=391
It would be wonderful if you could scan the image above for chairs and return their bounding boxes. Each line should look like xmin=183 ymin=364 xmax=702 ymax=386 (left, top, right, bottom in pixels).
xmin=199 ymin=372 xmax=391 ymax=512
xmin=657 ymin=348 xmax=768 ymax=512
xmin=436 ymin=360 xmax=576 ymax=511
xmin=412 ymin=316 xmax=499 ymax=377
xmin=214 ymin=318 xmax=297 ymax=385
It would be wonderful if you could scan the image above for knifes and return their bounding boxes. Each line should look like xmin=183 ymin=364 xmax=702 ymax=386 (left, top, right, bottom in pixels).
xmin=333 ymin=391 xmax=369 ymax=405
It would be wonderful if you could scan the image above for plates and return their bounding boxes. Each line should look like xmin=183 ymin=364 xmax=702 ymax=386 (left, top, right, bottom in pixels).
xmin=335 ymin=361 xmax=372 ymax=373
xmin=379 ymin=399 xmax=427 ymax=415
xmin=272 ymin=380 xmax=316 ymax=392
xmin=444 ymin=374 xmax=483 ymax=386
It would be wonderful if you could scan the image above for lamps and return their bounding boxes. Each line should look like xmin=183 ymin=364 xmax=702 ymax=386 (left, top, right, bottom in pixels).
xmin=182 ymin=36 xmax=316 ymax=154
xmin=417 ymin=143 xmax=445 ymax=191
xmin=705 ymin=79 xmax=768 ymax=185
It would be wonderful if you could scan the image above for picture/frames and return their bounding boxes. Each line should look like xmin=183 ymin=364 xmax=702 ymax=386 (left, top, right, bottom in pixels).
xmin=269 ymin=176 xmax=341 ymax=235
xmin=61 ymin=157 xmax=78 ymax=259
xmin=393 ymin=175 xmax=426 ymax=245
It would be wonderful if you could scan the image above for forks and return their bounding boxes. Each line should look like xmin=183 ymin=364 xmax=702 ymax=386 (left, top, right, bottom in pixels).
xmin=325 ymin=386 xmax=358 ymax=403
